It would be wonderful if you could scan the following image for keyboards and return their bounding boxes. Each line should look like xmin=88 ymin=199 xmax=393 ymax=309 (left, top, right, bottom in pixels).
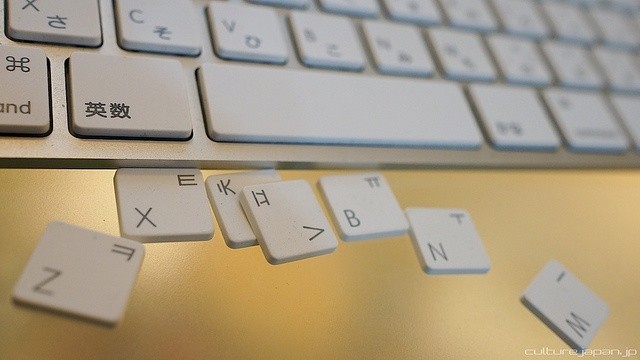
xmin=1 ymin=1 xmax=640 ymax=170
